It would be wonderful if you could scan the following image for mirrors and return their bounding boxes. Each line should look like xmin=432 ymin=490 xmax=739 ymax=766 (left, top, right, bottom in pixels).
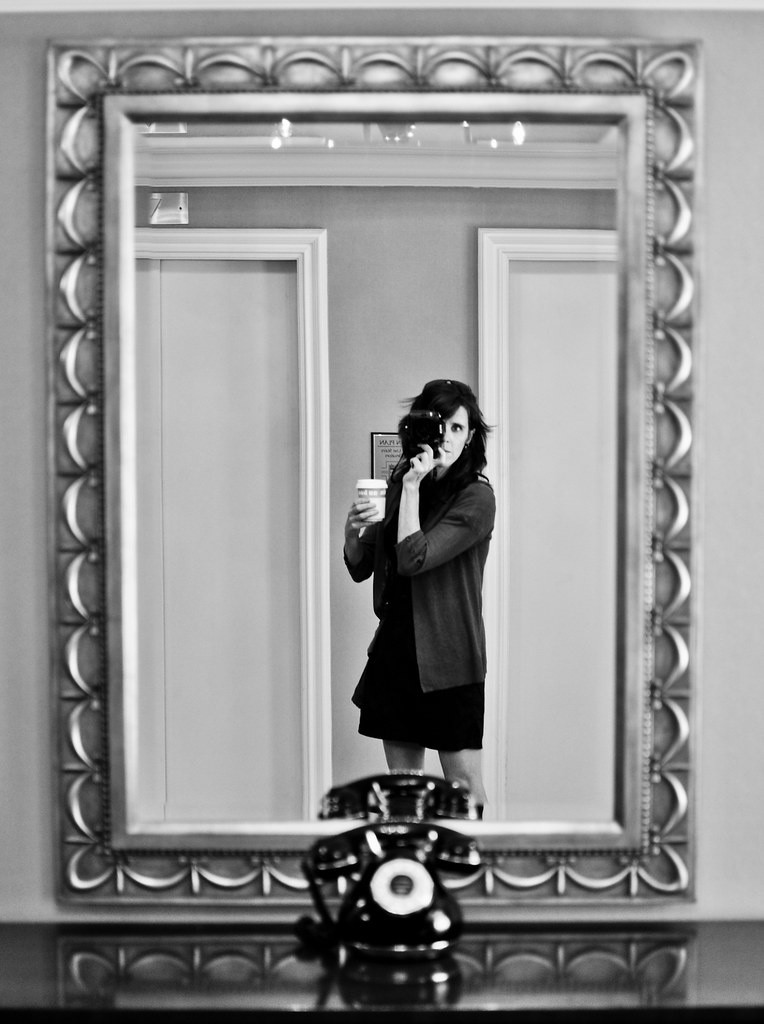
xmin=38 ymin=32 xmax=698 ymax=913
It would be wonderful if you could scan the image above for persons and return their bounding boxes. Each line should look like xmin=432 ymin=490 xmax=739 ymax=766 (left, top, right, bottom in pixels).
xmin=343 ymin=379 xmax=497 ymax=820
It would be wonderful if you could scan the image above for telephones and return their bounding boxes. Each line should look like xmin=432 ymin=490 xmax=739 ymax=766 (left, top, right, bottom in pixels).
xmin=297 ymin=823 xmax=485 ymax=963
xmin=319 ymin=774 xmax=481 ymax=821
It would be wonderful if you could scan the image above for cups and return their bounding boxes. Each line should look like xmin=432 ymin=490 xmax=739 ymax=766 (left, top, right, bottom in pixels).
xmin=356 ymin=480 xmax=388 ymax=521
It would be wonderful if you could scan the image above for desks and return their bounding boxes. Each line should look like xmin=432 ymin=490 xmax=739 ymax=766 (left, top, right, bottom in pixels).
xmin=0 ymin=921 xmax=764 ymax=1024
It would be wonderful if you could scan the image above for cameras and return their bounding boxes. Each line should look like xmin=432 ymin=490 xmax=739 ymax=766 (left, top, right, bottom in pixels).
xmin=397 ymin=411 xmax=445 ymax=447
